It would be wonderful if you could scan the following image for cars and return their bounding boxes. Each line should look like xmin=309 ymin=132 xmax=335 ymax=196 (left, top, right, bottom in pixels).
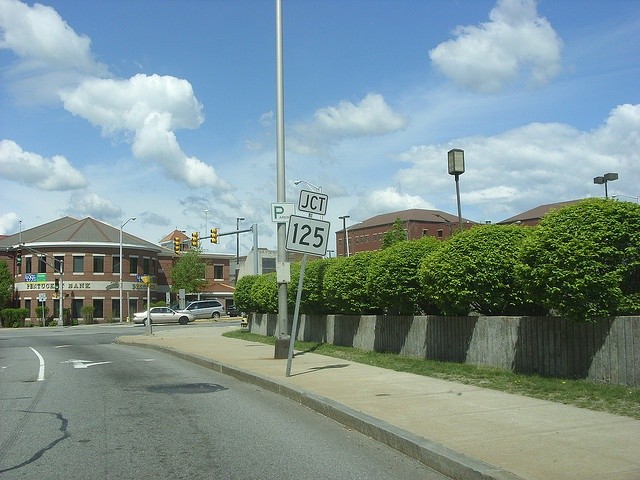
xmin=133 ymin=307 xmax=196 ymax=326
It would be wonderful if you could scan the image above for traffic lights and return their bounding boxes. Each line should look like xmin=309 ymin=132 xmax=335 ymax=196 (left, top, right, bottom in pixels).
xmin=140 ymin=275 xmax=150 ymax=286
xmin=174 ymin=237 xmax=180 ymax=255
xmin=192 ymin=232 xmax=198 ymax=247
xmin=15 ymin=249 xmax=22 ymax=266
xmin=55 ymin=278 xmax=59 ymax=298
xmin=211 ymin=229 xmax=217 ymax=244
xmin=120 ymin=216 xmax=136 ymax=325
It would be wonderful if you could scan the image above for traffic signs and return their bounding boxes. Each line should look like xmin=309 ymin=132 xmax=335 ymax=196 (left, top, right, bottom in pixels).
xmin=272 ymin=202 xmax=296 ymax=222
xmin=39 ymin=293 xmax=47 ymax=302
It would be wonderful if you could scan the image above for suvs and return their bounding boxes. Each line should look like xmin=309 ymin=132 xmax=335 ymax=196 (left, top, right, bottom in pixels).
xmin=226 ymin=305 xmax=248 ymax=317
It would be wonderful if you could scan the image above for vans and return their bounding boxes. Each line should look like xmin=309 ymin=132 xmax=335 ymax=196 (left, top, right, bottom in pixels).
xmin=175 ymin=301 xmax=224 ymax=319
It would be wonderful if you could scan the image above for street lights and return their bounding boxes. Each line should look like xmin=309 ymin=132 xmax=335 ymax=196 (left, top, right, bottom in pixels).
xmin=236 ymin=217 xmax=245 ymax=286
xmin=448 ymin=148 xmax=465 ymax=234
xmin=339 ymin=216 xmax=349 ymax=257
